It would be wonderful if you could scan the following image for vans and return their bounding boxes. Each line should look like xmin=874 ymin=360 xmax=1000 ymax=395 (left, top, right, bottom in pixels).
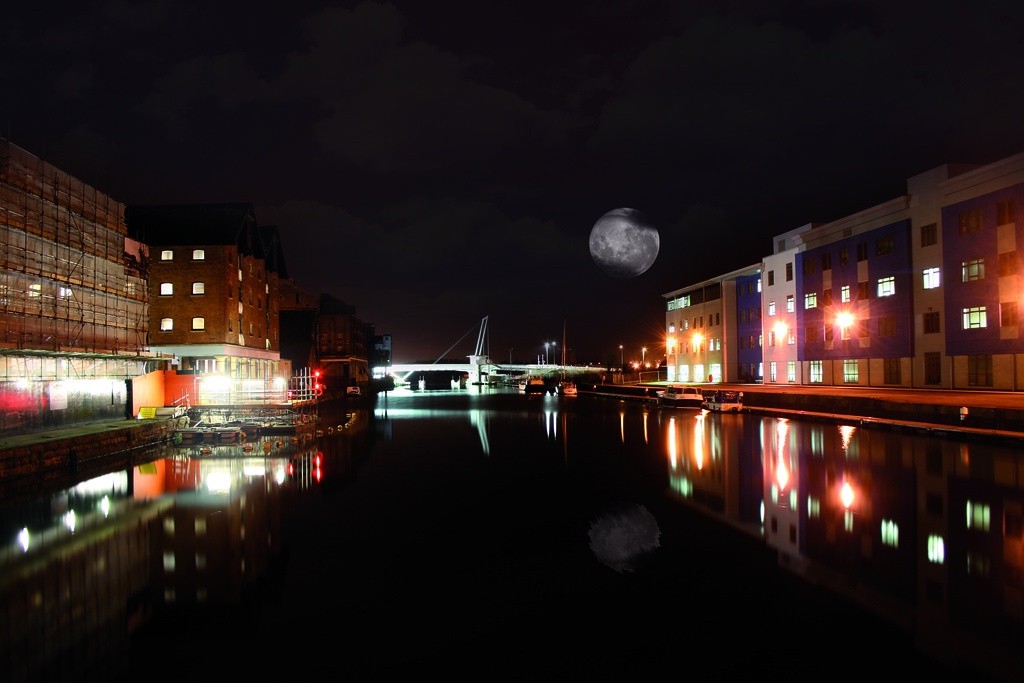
xmin=258 ymin=422 xmax=296 ymax=434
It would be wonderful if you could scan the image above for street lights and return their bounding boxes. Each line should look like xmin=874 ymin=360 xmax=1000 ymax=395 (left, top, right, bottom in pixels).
xmin=619 ymin=344 xmax=624 ymax=365
xmin=641 ymin=346 xmax=647 ymax=364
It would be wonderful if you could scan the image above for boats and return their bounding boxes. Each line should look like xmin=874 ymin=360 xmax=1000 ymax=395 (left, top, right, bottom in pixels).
xmin=524 ymin=374 xmax=546 ymax=396
xmin=663 ymin=384 xmax=704 ymax=406
xmin=705 ymin=390 xmax=743 ymax=413
xmin=554 ymin=381 xmax=578 ymax=395
